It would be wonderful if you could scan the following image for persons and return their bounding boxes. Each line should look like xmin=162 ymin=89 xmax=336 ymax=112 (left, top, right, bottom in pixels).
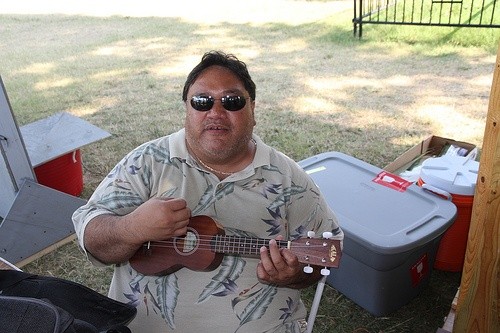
xmin=72 ymin=50 xmax=344 ymax=333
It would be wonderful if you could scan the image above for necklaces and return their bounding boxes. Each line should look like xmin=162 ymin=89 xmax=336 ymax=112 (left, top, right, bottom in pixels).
xmin=194 ymin=139 xmax=257 ymax=175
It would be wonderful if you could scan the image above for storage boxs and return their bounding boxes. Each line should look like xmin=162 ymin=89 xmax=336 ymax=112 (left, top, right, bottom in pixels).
xmin=382 ymin=135 xmax=477 ymax=179
xmin=296 ymin=151 xmax=458 ymax=316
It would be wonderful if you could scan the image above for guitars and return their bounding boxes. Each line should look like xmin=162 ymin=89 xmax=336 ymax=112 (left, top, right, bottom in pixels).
xmin=129 ymin=215 xmax=342 ymax=277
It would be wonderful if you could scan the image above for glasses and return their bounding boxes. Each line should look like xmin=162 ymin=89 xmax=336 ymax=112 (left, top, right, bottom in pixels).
xmin=185 ymin=94 xmax=252 ymax=112
